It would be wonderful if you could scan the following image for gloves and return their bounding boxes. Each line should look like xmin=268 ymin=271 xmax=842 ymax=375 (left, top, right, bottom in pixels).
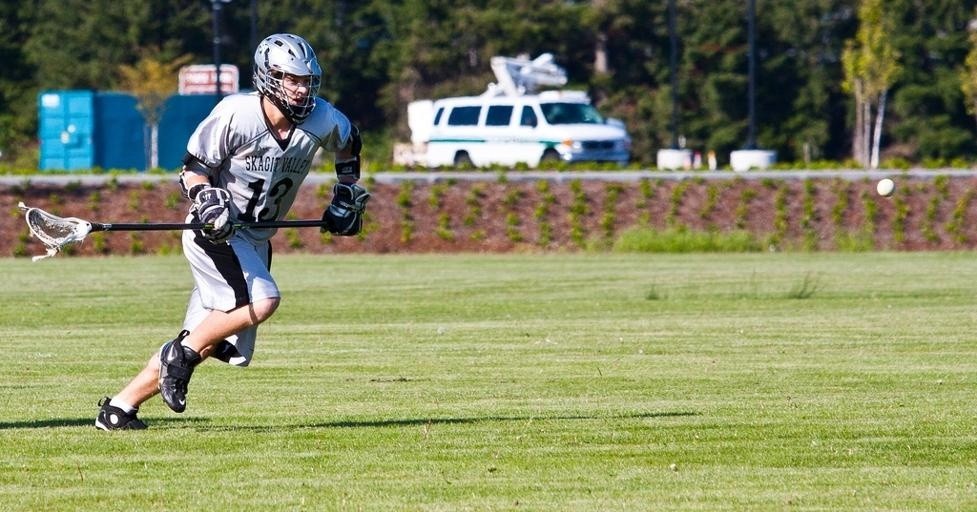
xmin=321 ymin=184 xmax=368 ymax=236
xmin=192 ymin=187 xmax=235 ymax=245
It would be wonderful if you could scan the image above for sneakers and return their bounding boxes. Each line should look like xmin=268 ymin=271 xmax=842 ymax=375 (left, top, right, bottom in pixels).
xmin=158 ymin=331 xmax=201 ymax=413
xmin=96 ymin=397 xmax=145 ymax=430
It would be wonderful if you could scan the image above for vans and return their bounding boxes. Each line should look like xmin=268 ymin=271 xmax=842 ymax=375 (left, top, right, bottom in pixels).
xmin=423 ymin=93 xmax=631 ymax=171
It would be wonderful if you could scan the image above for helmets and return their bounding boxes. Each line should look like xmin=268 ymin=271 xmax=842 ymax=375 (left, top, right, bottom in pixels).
xmin=253 ymin=35 xmax=322 ymax=125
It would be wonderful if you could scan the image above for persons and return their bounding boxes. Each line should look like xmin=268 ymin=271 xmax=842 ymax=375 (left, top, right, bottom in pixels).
xmin=93 ymin=32 xmax=371 ymax=430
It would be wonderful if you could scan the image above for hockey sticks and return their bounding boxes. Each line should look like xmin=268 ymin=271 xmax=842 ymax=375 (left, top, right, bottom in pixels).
xmin=18 ymin=201 xmax=322 ymax=262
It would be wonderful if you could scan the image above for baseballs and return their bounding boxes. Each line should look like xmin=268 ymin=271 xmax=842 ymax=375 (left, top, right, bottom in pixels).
xmin=875 ymin=177 xmax=896 ymax=198
